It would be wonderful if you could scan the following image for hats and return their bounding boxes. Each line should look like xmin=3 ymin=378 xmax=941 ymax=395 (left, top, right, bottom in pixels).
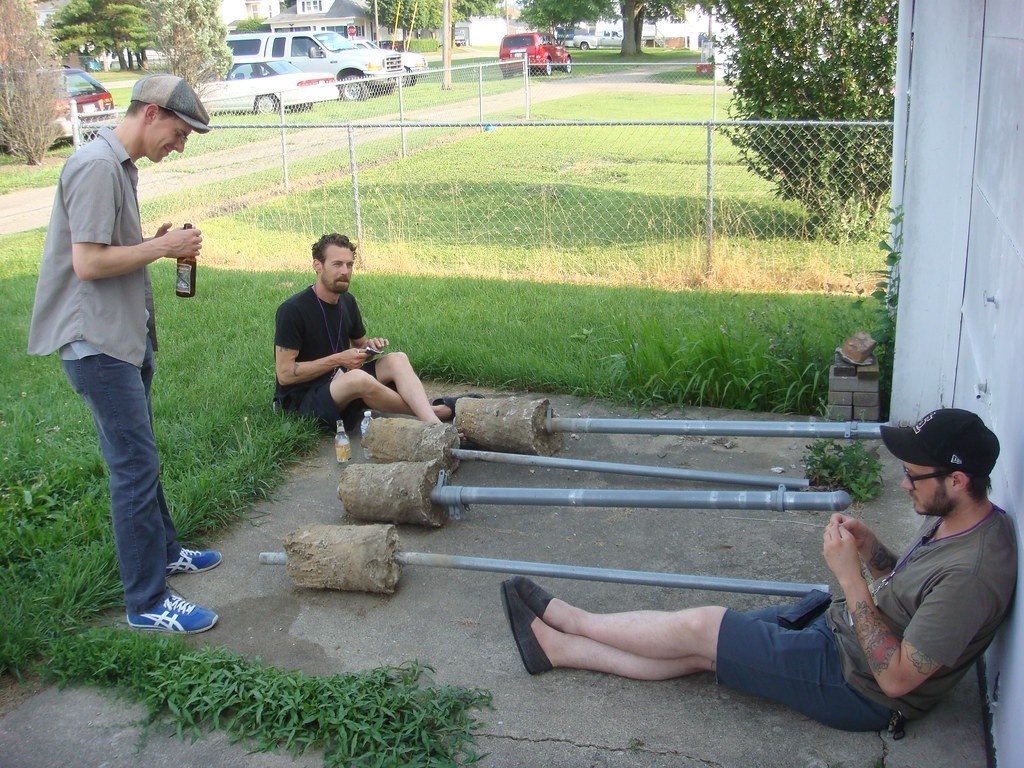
xmin=130 ymin=75 xmax=210 ymax=134
xmin=879 ymin=408 xmax=1000 ymax=475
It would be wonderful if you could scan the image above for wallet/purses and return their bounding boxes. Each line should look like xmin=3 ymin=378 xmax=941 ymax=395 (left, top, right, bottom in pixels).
xmin=776 ymin=588 xmax=833 ymax=629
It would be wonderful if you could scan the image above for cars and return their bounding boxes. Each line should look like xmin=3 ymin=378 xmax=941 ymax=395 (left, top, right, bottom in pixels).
xmin=573 ymin=28 xmax=622 ymax=49
xmin=186 ymin=58 xmax=340 ymax=116
xmin=18 ymin=66 xmax=114 ymax=124
xmin=501 ymin=32 xmax=573 ymax=78
xmin=352 ymin=37 xmax=431 ymax=86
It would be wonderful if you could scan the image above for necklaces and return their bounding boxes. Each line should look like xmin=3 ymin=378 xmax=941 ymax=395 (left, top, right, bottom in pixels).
xmin=312 ymin=286 xmax=343 ymax=353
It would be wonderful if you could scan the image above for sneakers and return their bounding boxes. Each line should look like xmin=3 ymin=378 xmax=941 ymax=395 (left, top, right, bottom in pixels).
xmin=513 ymin=576 xmax=555 ymax=619
xmin=166 ymin=546 xmax=222 ymax=576
xmin=127 ymin=595 xmax=219 ymax=634
xmin=500 ymin=580 xmax=555 ymax=675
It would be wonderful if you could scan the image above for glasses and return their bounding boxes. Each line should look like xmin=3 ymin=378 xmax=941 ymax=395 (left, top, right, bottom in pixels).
xmin=901 ymin=463 xmax=956 ymax=491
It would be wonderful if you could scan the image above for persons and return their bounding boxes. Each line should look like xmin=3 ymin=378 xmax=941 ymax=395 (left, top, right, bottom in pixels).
xmin=26 ymin=74 xmax=222 ymax=634
xmin=272 ymin=232 xmax=486 ymax=450
xmin=500 ymin=408 xmax=1018 ymax=731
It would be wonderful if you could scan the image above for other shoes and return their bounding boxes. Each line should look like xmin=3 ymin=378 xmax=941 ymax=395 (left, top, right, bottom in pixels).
xmin=433 ymin=394 xmax=487 ymax=420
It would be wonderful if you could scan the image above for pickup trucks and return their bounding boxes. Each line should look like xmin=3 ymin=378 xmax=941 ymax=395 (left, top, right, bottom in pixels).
xmin=226 ymin=29 xmax=404 ymax=102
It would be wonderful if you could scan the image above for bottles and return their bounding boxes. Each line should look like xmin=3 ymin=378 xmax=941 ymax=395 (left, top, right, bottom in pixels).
xmin=360 ymin=409 xmax=376 ymax=460
xmin=334 ymin=419 xmax=353 ymax=466
xmin=175 ymin=221 xmax=198 ymax=299
